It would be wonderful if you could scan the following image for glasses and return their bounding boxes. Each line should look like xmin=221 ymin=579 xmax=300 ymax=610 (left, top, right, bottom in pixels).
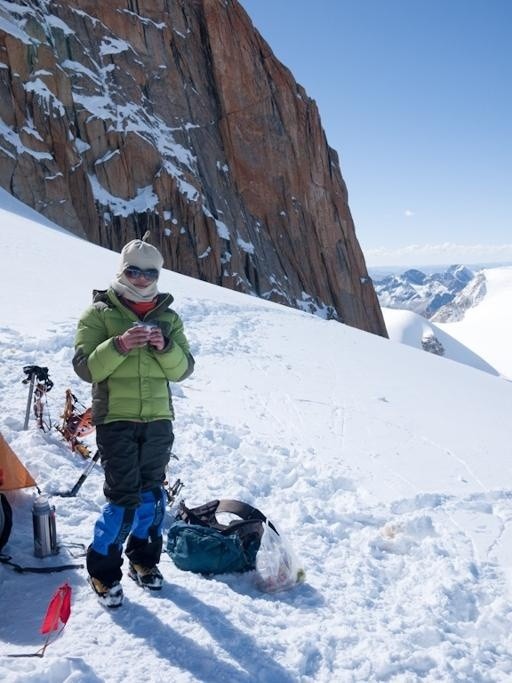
xmin=125 ymin=264 xmax=159 ymax=282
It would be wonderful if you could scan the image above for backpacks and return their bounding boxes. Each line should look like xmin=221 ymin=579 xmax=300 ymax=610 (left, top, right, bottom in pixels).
xmin=164 ymin=498 xmax=281 ymax=580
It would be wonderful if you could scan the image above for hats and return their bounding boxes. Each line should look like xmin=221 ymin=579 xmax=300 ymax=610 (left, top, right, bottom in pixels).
xmin=109 ymin=230 xmax=164 ymax=304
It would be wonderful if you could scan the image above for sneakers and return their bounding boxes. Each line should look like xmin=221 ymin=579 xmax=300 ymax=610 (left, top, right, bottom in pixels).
xmin=127 ymin=558 xmax=166 ymax=593
xmin=84 ymin=573 xmax=125 ymax=611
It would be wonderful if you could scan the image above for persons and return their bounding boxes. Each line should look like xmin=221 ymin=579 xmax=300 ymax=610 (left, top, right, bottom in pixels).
xmin=73 ymin=239 xmax=196 ymax=607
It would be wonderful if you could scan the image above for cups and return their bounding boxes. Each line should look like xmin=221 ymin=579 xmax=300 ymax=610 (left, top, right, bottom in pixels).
xmin=32 ymin=497 xmax=58 ymax=557
xmin=134 ymin=321 xmax=158 ymax=341
